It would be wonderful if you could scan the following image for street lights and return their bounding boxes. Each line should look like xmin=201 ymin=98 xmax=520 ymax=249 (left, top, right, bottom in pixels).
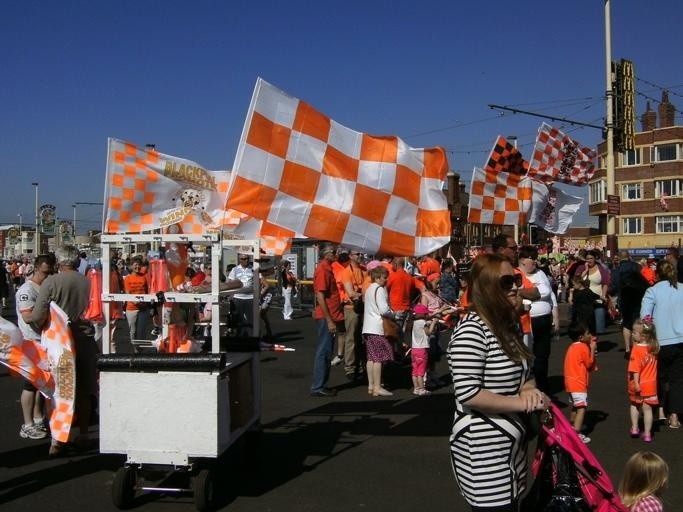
xmin=71 ymin=205 xmax=77 ymax=237
xmin=144 ymin=143 xmax=156 ymax=150
xmin=16 ymin=212 xmax=22 ymax=236
xmin=31 ymin=181 xmax=40 ymax=257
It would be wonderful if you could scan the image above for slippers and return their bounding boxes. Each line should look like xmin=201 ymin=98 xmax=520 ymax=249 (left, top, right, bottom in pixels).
xmin=656 ymin=418 xmax=682 ymax=429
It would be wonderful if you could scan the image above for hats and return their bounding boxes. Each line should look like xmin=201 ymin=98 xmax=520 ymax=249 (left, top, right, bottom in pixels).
xmin=415 ymin=304 xmax=433 ymax=314
xmin=427 ymin=273 xmax=440 ymax=281
xmin=367 ymin=260 xmax=382 ymax=273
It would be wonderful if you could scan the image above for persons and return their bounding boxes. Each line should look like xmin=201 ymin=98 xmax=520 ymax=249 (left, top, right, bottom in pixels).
xmin=281 ymin=261 xmax=297 ymax=320
xmin=310 ymin=234 xmax=682 ymax=511
xmin=1 ymin=246 xmax=212 ymax=454
xmin=219 ymin=251 xmax=273 ymax=342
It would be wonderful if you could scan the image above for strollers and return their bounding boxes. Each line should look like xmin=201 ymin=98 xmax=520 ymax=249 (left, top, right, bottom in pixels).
xmin=508 ymin=395 xmax=629 ymax=512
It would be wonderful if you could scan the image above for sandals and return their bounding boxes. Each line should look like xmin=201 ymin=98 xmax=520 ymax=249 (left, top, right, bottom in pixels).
xmin=413 ymin=388 xmax=433 ymax=396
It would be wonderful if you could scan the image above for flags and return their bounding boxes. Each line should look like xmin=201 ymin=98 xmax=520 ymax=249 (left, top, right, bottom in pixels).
xmin=223 ymin=77 xmax=451 ymax=257
xmin=526 ymin=177 xmax=584 ymax=233
xmin=102 ymin=138 xmax=295 ymax=257
xmin=484 ymin=135 xmax=530 ymax=176
xmin=467 ymin=166 xmax=532 ymax=225
xmin=530 ymin=122 xmax=597 ymax=187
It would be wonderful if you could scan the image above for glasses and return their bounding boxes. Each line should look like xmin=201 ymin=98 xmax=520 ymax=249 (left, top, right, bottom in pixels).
xmin=497 ymin=273 xmax=522 ymax=290
xmin=241 ymin=257 xmax=249 ymax=261
xmin=351 ymin=252 xmax=364 ymax=256
xmin=505 ymin=245 xmax=518 ymax=251
xmin=322 ymin=249 xmax=336 ymax=256
xmin=528 ymin=257 xmax=538 ymax=260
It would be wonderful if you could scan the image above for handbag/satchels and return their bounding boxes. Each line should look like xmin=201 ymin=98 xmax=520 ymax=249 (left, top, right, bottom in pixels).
xmin=582 ymin=279 xmax=591 ymax=288
xmin=383 ymin=317 xmax=399 ymax=342
xmin=606 ymin=298 xmax=620 ymax=320
xmin=520 ymin=483 xmax=593 ymax=512
xmin=620 ymin=270 xmax=646 ymax=292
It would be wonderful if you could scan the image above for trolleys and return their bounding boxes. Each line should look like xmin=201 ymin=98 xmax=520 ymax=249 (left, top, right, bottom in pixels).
xmin=93 ymin=231 xmax=270 ymax=512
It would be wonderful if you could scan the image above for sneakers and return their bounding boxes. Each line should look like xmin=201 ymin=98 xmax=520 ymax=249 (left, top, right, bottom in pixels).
xmin=34 ymin=416 xmax=50 ymax=431
xmin=347 ymin=372 xmax=356 ymax=380
xmin=577 ymin=434 xmax=591 ymax=443
xmin=20 ymin=424 xmax=47 ymax=440
xmin=629 ymin=426 xmax=640 ymax=436
xmin=641 ymin=432 xmax=653 ymax=442
xmin=331 ymin=355 xmax=344 ymax=367
xmin=49 ymin=436 xmax=91 ymax=457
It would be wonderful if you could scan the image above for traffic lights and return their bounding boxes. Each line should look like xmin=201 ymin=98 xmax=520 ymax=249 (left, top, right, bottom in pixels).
xmin=546 ymin=239 xmax=552 ymax=253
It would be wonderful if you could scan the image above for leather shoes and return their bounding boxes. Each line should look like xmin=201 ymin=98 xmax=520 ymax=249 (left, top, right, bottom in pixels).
xmin=367 ymin=383 xmax=394 ymax=397
xmin=312 ymin=386 xmax=336 ymax=396
